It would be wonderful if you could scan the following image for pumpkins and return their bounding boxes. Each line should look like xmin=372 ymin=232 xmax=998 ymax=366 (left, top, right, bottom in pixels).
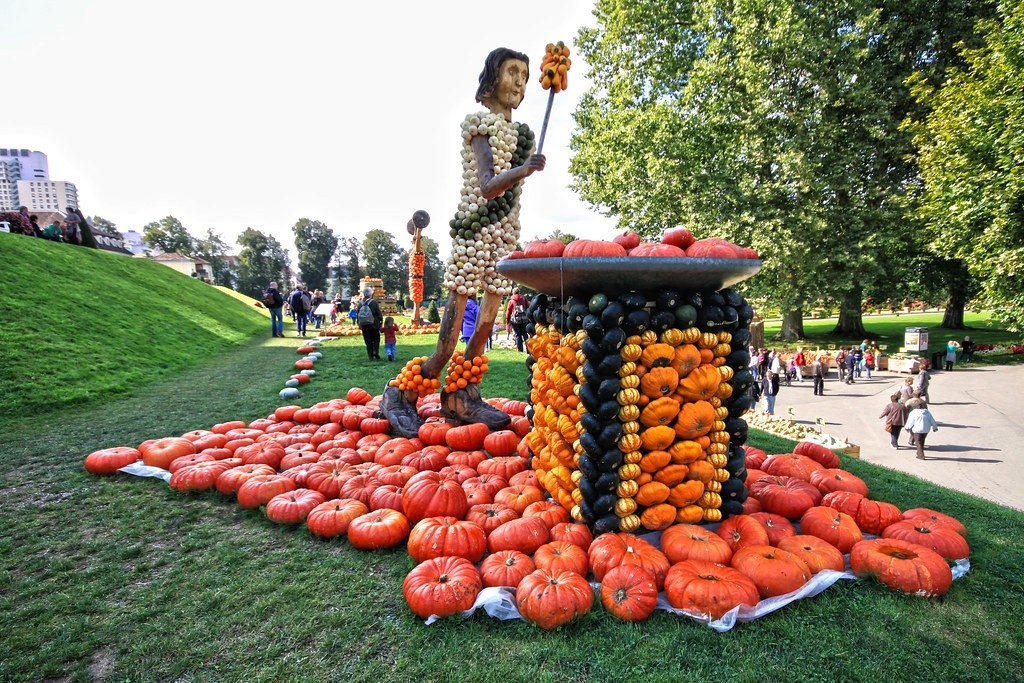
xmin=539 ymin=41 xmax=571 ymax=93
xmin=412 ymin=210 xmax=430 ymax=228
xmin=512 ymin=287 xmax=755 ymax=537
xmin=739 ymin=408 xmax=852 ymax=449
xmin=803 ymin=351 xmax=829 ymax=365
xmin=319 ymin=315 xmax=363 ymax=336
xmin=508 ymin=227 xmax=759 ymax=259
xmin=407 ymin=219 xmax=417 ymax=235
xmin=409 ymin=249 xmax=425 ymax=302
xmin=662 ymin=442 xmax=968 ymax=622
xmin=85 ymin=386 xmax=671 ymax=625
xmin=442 ymin=112 xmax=538 ymax=295
xmin=285 ymin=339 xmax=322 ymax=387
xmin=392 ymin=322 xmax=441 ymax=336
xmin=359 ymin=276 xmax=386 ymax=297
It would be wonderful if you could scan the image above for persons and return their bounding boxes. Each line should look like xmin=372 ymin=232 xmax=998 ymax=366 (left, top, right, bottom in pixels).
xmin=879 ymin=365 xmax=939 ymax=461
xmin=290 ymin=284 xmax=312 ymax=337
xmin=961 ymin=335 xmax=975 ymax=363
xmin=747 ymin=340 xmax=882 ymax=415
xmin=379 ymin=47 xmax=546 ymax=437
xmin=329 ymin=293 xmax=361 ymax=327
xmin=408 ymin=226 xmax=425 ymax=321
xmin=946 ymin=340 xmax=961 ymax=371
xmin=460 ymin=286 xmax=531 ymax=355
xmin=279 ymin=286 xmax=326 ymax=328
xmin=356 ymin=287 xmax=383 ymax=360
xmin=379 ymin=316 xmax=400 ymax=361
xmin=264 ymin=282 xmax=286 ymax=339
xmin=18 ymin=205 xmax=82 ymax=244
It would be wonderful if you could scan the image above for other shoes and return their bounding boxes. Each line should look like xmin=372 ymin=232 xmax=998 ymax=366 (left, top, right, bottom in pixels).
xmin=915 ymin=454 xmax=918 ymax=458
xmin=374 ymin=353 xmax=380 ymax=359
xmin=302 ymin=332 xmax=306 ymax=336
xmin=846 ymin=382 xmax=851 ymax=385
xmin=388 ymin=355 xmax=392 ymax=361
xmin=273 ymin=334 xmax=278 ymax=337
xmin=370 ymin=357 xmax=373 ymax=359
xmin=278 ymin=333 xmax=284 ymax=338
xmin=297 ymin=332 xmax=300 ymax=336
xmin=894 ymin=445 xmax=898 ymax=449
xmin=486 ymin=348 xmax=488 ymax=352
xmin=851 ymin=381 xmax=855 ymax=383
xmin=908 ymin=442 xmax=914 ymax=446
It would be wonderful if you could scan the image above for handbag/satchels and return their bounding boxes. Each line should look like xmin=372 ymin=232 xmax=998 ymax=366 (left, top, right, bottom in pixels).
xmin=301 ymin=291 xmax=311 ymax=310
xmin=65 ymin=226 xmax=73 ymax=234
xmin=886 ymin=420 xmax=892 ymax=432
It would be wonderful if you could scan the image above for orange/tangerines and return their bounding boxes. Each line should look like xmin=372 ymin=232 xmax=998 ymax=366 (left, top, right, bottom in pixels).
xmin=388 ymin=350 xmax=488 ymax=398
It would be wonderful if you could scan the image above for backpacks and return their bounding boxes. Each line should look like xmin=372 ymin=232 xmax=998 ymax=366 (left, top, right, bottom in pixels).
xmin=358 ymin=299 xmax=374 ymax=326
xmin=514 ymin=305 xmax=528 ymax=323
xmin=261 ymin=289 xmax=274 ymax=304
